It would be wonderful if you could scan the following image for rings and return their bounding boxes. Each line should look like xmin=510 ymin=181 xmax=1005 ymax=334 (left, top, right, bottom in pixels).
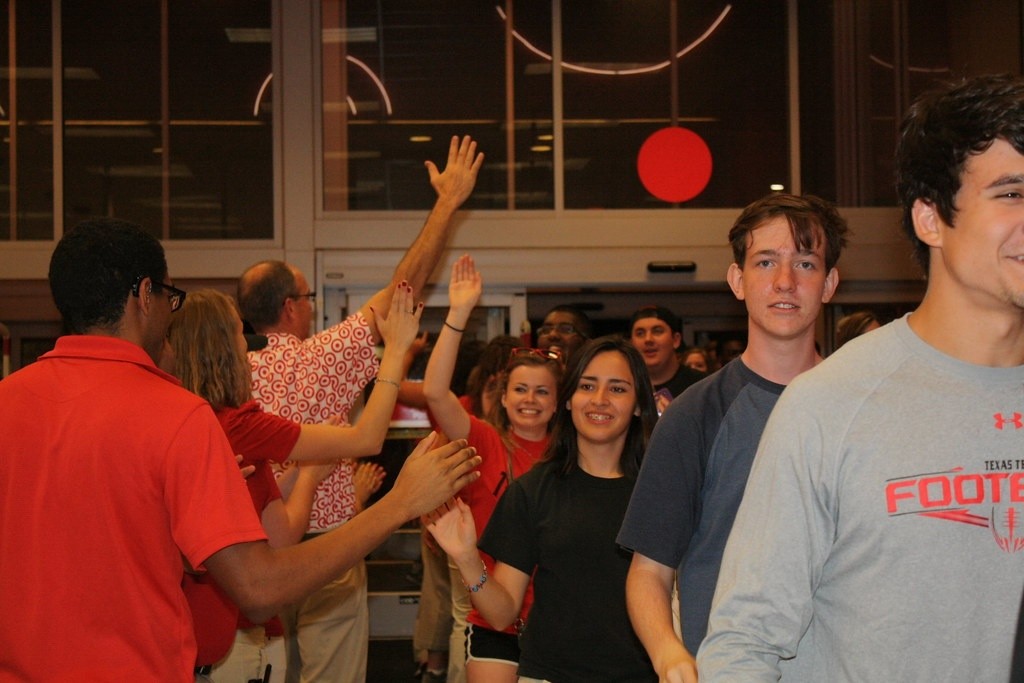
xmin=406 ymin=310 xmax=413 ymax=313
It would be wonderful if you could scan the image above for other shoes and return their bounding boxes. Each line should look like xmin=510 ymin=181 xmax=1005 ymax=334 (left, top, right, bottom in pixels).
xmin=421 ymin=671 xmax=447 ymax=683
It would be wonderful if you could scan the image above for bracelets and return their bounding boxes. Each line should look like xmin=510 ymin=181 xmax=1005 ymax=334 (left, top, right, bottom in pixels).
xmin=445 ymin=321 xmax=465 ymax=332
xmin=376 ymin=378 xmax=398 ymax=387
xmin=463 ymin=561 xmax=487 ymax=591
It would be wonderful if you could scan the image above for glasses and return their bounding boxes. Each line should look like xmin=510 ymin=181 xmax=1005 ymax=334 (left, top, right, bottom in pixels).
xmin=539 ymin=323 xmax=587 ymax=340
xmin=507 ymin=347 xmax=565 ymax=376
xmin=282 ymin=290 xmax=317 ymax=307
xmin=132 ymin=276 xmax=186 ymax=312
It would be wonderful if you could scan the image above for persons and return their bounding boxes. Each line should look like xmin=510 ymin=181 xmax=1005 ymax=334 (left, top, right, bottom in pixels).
xmin=353 ymin=462 xmax=387 ymax=514
xmin=157 ymin=279 xmax=423 ymax=683
xmin=617 ymin=192 xmax=848 ymax=682
xmin=715 ymin=334 xmax=747 ymax=367
xmin=684 ymin=346 xmax=714 ymax=376
xmin=420 ymin=334 xmax=659 ymax=683
xmin=537 ymin=303 xmax=590 ymax=367
xmin=0 ymin=216 xmax=482 ymax=682
xmin=631 ymin=306 xmax=710 ymax=416
xmin=238 ymin=135 xmax=485 ymax=682
xmin=398 ymin=254 xmax=568 ymax=683
xmin=696 ymin=69 xmax=1024 ymax=683
xmin=835 ymin=311 xmax=880 ymax=348
xmin=246 ymin=460 xmax=335 ymax=682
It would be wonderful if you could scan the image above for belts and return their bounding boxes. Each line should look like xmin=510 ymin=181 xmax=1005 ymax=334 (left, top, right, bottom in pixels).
xmin=302 ymin=533 xmax=326 ymax=542
